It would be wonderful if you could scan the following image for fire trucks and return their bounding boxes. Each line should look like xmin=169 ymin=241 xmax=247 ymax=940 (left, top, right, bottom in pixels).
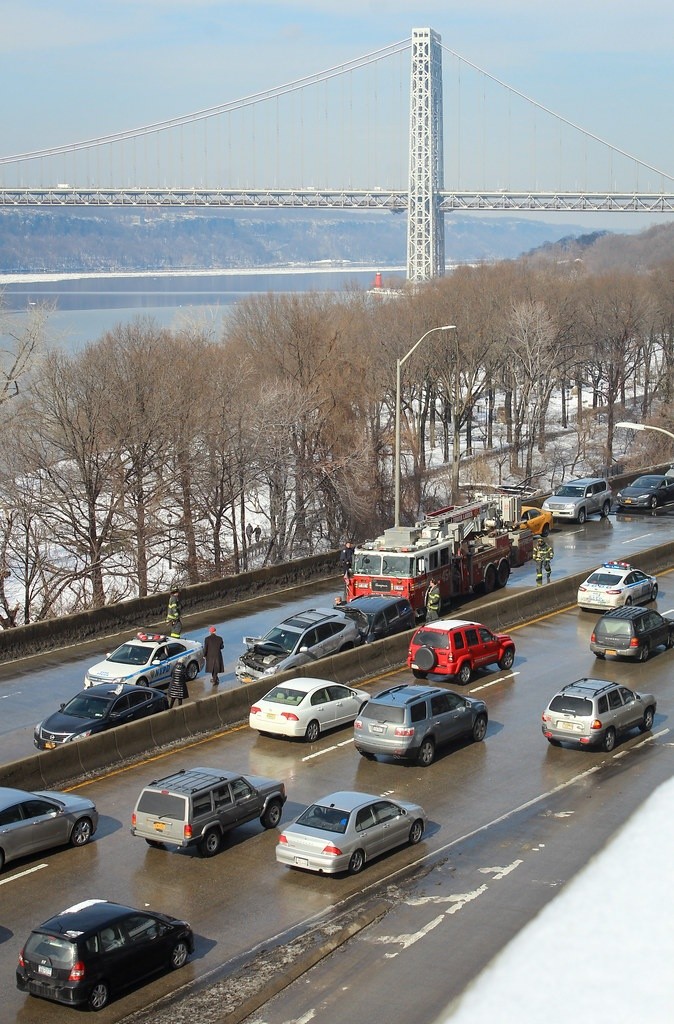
xmin=341 ymin=499 xmax=534 ymax=627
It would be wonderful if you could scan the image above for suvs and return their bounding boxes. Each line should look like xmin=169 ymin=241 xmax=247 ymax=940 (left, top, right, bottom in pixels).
xmin=130 ymin=766 xmax=287 ymax=858
xmin=235 ymin=608 xmax=362 ymax=683
xmin=590 ymin=605 xmax=674 ymax=663
xmin=333 ymin=594 xmax=417 ymax=645
xmin=352 ymin=684 xmax=489 ymax=768
xmin=542 ymin=476 xmax=614 ymax=525
xmin=542 ymin=677 xmax=658 ymax=753
xmin=406 ymin=618 xmax=516 ymax=686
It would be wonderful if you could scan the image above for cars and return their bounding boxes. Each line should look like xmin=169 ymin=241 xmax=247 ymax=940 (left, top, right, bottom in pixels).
xmin=520 ymin=505 xmax=554 ymax=538
xmin=616 ymin=474 xmax=674 ymax=510
xmin=274 ymin=791 xmax=428 ymax=875
xmin=84 ymin=632 xmax=205 ymax=693
xmin=33 ymin=682 xmax=170 ymax=751
xmin=15 ymin=897 xmax=196 ymax=1011
xmin=578 ymin=560 xmax=658 ymax=614
xmin=250 ymin=677 xmax=372 ymax=743
xmin=0 ymin=786 xmax=99 ymax=871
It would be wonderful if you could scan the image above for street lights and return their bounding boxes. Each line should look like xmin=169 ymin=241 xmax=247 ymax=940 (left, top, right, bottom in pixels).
xmin=167 ymin=512 xmax=172 ymax=569
xmin=485 ymin=397 xmax=488 ymax=438
xmin=395 ymin=325 xmax=457 ymax=526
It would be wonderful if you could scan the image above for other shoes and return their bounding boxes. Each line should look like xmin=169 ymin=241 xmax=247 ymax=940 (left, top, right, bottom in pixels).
xmin=210 ymin=678 xmax=219 ymax=682
xmin=547 ymin=574 xmax=550 ymax=577
xmin=213 ymin=681 xmax=218 ymax=686
xmin=536 ymin=577 xmax=542 ymax=581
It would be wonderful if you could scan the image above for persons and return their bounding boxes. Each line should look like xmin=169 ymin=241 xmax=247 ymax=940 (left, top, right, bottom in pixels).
xmin=340 ymin=542 xmax=353 ymax=575
xmin=533 ymin=537 xmax=553 ymax=581
xmin=246 ymin=523 xmax=253 ymax=545
xmin=252 ymin=525 xmax=261 ymax=542
xmin=168 ymin=657 xmax=189 ymax=709
xmin=165 ymin=587 xmax=182 ymax=639
xmin=335 ymin=596 xmax=347 ymax=605
xmin=203 ymin=627 xmax=224 ymax=685
xmin=426 ymin=579 xmax=440 ymax=623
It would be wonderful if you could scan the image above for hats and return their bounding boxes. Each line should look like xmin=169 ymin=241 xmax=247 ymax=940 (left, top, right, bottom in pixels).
xmin=209 ymin=627 xmax=216 ymax=632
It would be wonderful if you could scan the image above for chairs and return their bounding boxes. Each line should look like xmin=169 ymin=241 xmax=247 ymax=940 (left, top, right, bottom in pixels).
xmin=276 ymin=692 xmax=303 ymax=702
xmin=101 ymin=928 xmax=115 ymax=949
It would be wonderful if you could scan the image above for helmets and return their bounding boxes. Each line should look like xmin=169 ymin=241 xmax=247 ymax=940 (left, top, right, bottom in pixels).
xmin=538 ymin=538 xmax=543 ymax=542
xmin=170 ymin=585 xmax=180 ymax=593
